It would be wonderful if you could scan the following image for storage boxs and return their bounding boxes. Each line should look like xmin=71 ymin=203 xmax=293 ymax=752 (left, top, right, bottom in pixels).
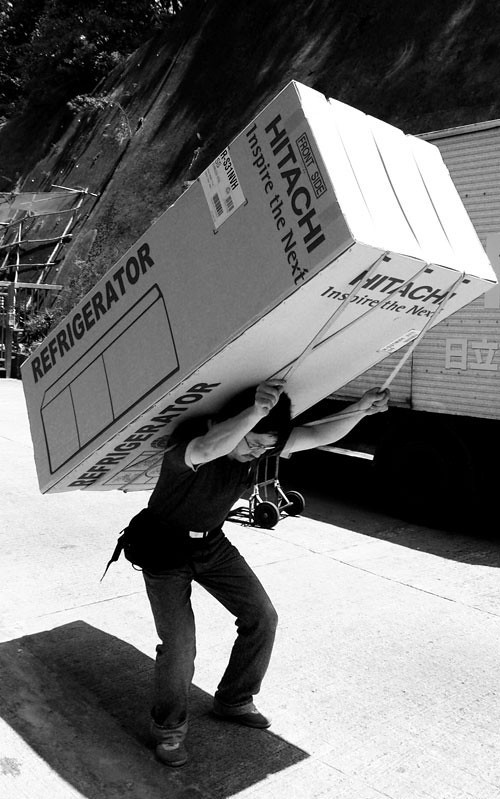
xmin=18 ymin=81 xmax=499 ymax=490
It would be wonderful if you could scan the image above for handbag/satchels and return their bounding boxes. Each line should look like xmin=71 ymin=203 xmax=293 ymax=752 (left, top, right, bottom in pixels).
xmin=107 ymin=510 xmax=194 ymax=572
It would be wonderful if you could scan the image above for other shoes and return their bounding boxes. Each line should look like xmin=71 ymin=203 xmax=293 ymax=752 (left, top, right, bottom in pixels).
xmin=217 ymin=706 xmax=271 ymax=730
xmin=156 ymin=739 xmax=189 ymax=766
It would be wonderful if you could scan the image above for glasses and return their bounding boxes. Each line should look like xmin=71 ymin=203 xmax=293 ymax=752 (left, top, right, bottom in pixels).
xmin=245 ymin=439 xmax=277 ymax=454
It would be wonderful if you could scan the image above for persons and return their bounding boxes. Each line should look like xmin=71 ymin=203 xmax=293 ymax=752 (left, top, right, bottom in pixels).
xmin=124 ymin=377 xmax=391 ymax=766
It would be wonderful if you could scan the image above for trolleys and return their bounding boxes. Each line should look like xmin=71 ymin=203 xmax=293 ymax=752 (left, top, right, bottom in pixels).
xmin=226 ymin=450 xmax=305 ymax=528
